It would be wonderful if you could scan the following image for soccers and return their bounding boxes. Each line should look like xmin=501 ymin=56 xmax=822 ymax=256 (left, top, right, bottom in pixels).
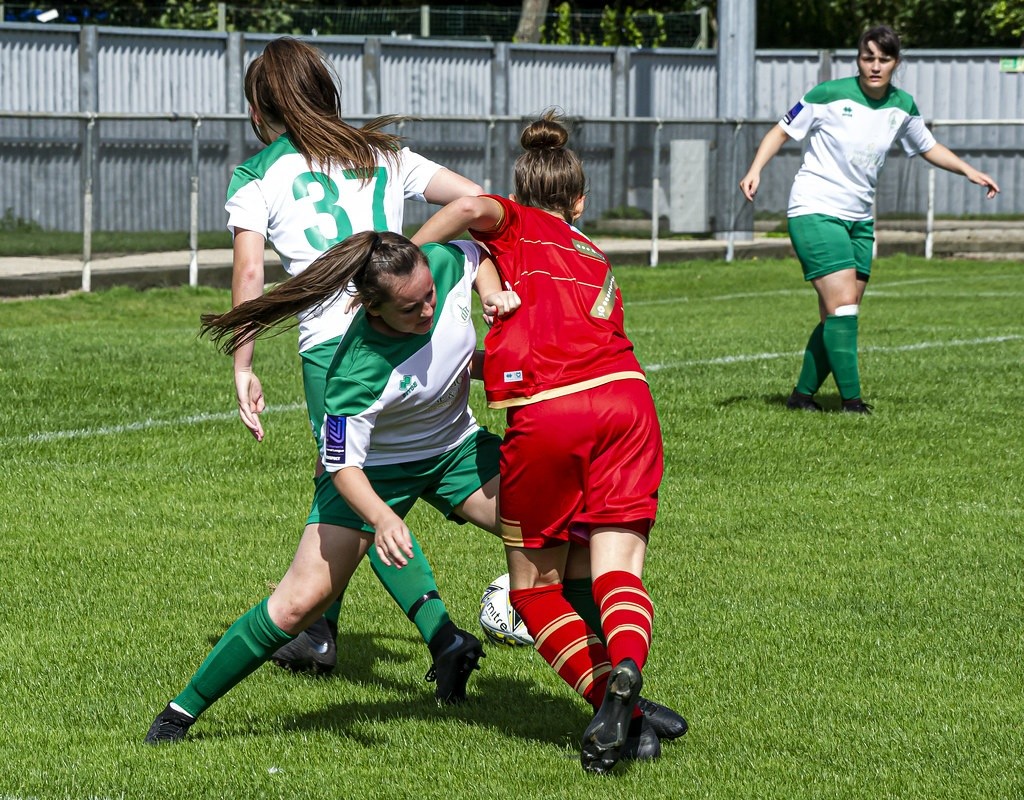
xmin=479 ymin=573 xmax=537 ymax=648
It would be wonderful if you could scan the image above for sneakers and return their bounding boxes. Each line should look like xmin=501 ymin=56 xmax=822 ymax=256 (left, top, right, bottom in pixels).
xmin=788 ymin=388 xmax=825 ymax=412
xmin=838 ymin=398 xmax=874 ymax=416
xmin=264 ymin=619 xmax=339 ymax=673
xmin=143 ymin=704 xmax=196 ymax=746
xmin=632 ymin=694 xmax=688 ymax=740
xmin=428 ymin=622 xmax=487 ymax=704
xmin=578 ymin=659 xmax=663 ymax=773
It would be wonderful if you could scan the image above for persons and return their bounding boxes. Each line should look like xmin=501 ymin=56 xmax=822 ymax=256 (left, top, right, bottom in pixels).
xmin=231 ymin=34 xmax=490 ymax=707
xmin=144 ymin=231 xmax=692 ymax=750
xmin=737 ymin=25 xmax=1003 ymax=416
xmin=344 ymin=105 xmax=661 ymax=776
